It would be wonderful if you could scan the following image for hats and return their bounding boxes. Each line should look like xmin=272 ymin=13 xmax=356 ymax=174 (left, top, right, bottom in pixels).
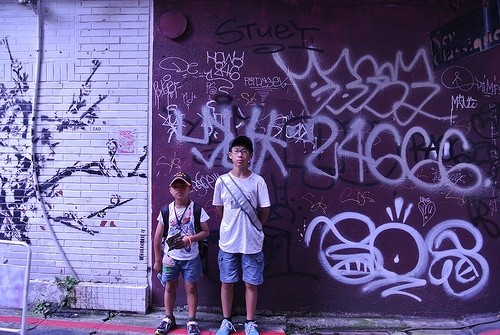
xmin=168 ymin=172 xmax=192 ymax=188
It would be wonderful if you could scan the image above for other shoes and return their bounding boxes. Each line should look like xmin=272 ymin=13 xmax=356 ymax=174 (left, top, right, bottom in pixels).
xmin=185 ymin=318 xmax=200 ymax=335
xmin=154 ymin=317 xmax=176 ymax=335
xmin=215 ymin=319 xmax=237 ymax=335
xmin=243 ymin=321 xmax=259 ymax=335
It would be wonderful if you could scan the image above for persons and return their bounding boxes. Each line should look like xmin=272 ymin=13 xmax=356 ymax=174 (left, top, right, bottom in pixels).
xmin=212 ymin=136 xmax=269 ymax=335
xmin=153 ymin=172 xmax=210 ymax=335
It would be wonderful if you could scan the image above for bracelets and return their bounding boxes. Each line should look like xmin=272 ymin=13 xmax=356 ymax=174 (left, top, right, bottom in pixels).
xmin=185 ymin=236 xmax=192 ymax=247
